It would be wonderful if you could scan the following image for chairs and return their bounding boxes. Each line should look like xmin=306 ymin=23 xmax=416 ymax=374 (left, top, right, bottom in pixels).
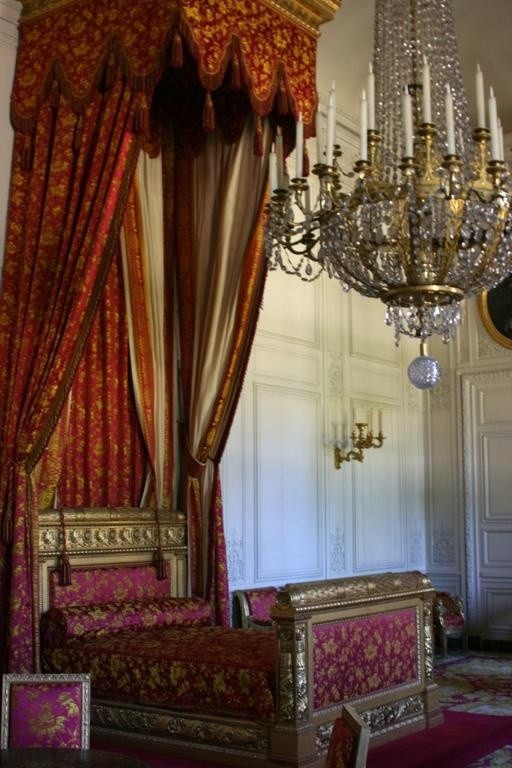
xmin=0 ymin=668 xmax=92 ymax=750
xmin=321 ymin=701 xmax=374 ymax=768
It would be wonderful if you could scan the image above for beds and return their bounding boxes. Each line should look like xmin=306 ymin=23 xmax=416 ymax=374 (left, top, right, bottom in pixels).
xmin=30 ymin=505 xmax=445 ymax=761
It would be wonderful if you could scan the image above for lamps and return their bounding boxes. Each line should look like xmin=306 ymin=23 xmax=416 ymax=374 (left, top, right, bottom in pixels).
xmin=265 ymin=0 xmax=511 ymax=390
